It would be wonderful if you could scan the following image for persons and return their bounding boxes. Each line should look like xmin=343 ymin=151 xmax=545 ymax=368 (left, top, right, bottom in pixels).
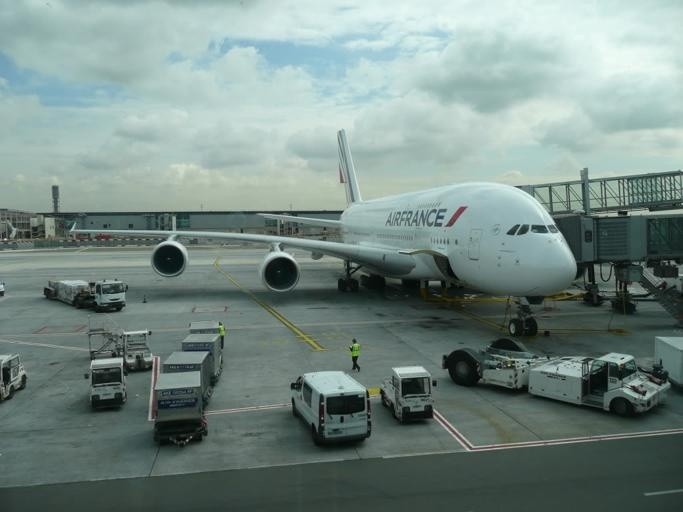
xmin=217 ymin=321 xmax=225 ymax=350
xmin=349 ymin=338 xmax=360 ymax=373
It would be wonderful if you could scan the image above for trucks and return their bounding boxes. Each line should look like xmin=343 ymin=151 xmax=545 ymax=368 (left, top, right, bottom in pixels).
xmin=289 ymin=370 xmax=370 ymax=446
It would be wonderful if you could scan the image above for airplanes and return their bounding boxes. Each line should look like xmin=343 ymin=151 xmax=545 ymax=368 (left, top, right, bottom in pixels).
xmin=68 ymin=127 xmax=577 ymax=337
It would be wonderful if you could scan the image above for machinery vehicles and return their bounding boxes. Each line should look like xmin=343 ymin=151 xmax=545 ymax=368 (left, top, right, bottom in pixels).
xmin=43 ymin=278 xmax=128 ymax=313
xmin=441 ymin=338 xmax=670 ymax=418
